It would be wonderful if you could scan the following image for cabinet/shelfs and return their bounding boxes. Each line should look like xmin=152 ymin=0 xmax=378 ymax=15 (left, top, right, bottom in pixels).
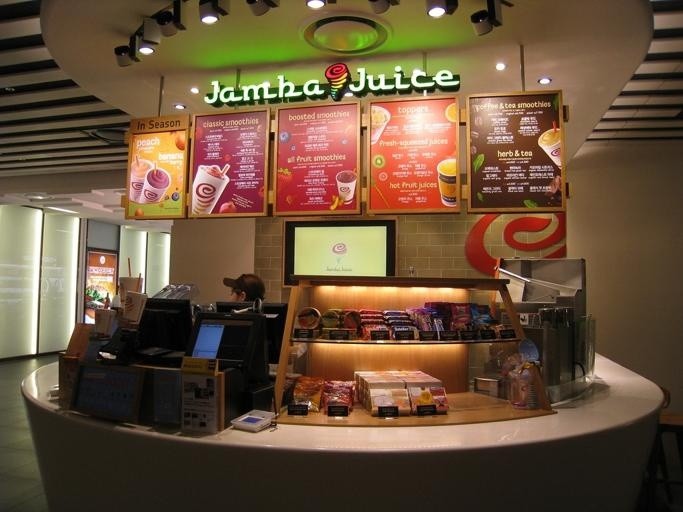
xmin=269 ymin=273 xmax=558 ymax=427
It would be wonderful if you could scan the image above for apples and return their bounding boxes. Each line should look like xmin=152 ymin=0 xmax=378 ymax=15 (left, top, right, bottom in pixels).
xmin=219 ymin=203 xmax=236 ymax=213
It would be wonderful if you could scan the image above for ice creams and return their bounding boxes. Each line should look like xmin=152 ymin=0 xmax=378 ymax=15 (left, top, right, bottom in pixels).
xmin=325 ymin=63 xmax=351 ymax=102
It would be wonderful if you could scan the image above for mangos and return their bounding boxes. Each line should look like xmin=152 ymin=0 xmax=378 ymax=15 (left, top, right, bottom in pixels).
xmin=175 ymin=135 xmax=185 ymax=150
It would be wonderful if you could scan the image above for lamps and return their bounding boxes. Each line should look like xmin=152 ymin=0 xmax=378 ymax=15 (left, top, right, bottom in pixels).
xmin=115 ymin=0 xmax=504 ymax=69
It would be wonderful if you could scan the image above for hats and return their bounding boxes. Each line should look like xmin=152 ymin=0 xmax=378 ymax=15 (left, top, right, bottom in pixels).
xmin=221 ymin=272 xmax=266 ymax=298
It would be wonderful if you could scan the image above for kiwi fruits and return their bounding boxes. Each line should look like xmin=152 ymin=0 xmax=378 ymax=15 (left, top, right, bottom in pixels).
xmin=373 ymin=155 xmax=385 ymax=168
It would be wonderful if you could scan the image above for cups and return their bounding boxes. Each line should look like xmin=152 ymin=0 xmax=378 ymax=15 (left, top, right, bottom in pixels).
xmin=536 ymin=128 xmax=561 ymax=171
xmin=117 ymin=276 xmax=148 ymax=326
xmin=190 ymin=164 xmax=230 ymax=215
xmin=435 ymin=158 xmax=456 ymax=208
xmin=93 ymin=308 xmax=117 ymax=339
xmin=334 ymin=169 xmax=357 ymax=205
xmin=369 ymin=105 xmax=391 ymax=145
xmin=128 ymin=158 xmax=171 ymax=204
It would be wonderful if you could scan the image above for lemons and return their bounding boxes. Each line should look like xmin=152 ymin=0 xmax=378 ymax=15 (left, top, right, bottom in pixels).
xmin=445 ymin=103 xmax=457 ymax=122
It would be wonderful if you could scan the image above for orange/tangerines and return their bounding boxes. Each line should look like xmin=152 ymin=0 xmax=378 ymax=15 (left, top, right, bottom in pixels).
xmin=135 ymin=208 xmax=144 ymax=216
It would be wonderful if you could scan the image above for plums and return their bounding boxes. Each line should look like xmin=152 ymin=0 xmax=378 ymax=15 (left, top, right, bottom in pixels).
xmin=171 ymin=192 xmax=179 ymax=200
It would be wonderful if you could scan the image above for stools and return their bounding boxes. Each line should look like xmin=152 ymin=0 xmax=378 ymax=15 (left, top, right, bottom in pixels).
xmin=650 ymin=386 xmax=683 ymax=506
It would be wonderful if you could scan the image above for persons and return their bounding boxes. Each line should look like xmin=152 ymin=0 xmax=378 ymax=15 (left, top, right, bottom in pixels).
xmin=221 ymin=274 xmax=266 ymax=304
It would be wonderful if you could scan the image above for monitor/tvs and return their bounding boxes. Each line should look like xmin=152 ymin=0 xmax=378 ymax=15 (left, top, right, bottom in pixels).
xmin=138 ymin=298 xmax=190 ymax=357
xmin=185 ymin=313 xmax=262 ymax=374
xmin=284 ymin=220 xmax=395 ymax=284
xmin=216 ymin=302 xmax=288 ymax=364
